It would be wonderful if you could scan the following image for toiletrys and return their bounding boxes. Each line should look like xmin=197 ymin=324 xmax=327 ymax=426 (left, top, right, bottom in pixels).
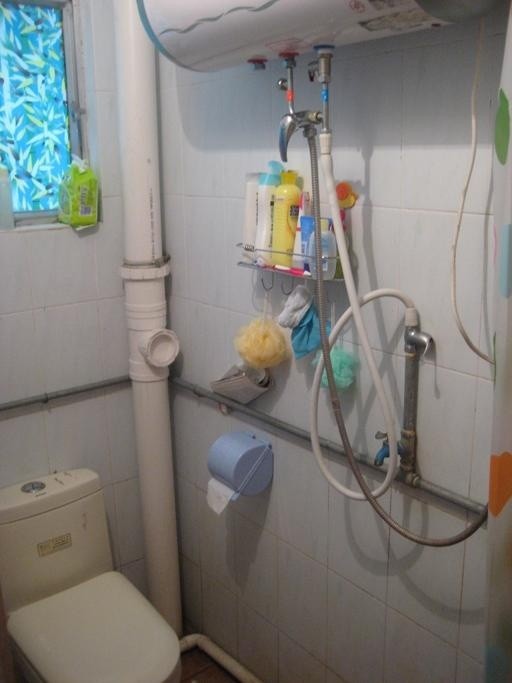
xmin=297 ymin=213 xmax=333 ymax=271
xmin=307 ymin=217 xmax=338 ymax=280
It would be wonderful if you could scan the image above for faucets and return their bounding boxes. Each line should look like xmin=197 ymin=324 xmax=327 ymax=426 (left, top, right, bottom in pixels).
xmin=373 ymin=442 xmax=390 ymax=468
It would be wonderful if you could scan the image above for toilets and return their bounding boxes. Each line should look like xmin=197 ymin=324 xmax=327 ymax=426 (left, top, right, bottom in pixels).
xmin=1 ymin=466 xmax=180 ymax=682
